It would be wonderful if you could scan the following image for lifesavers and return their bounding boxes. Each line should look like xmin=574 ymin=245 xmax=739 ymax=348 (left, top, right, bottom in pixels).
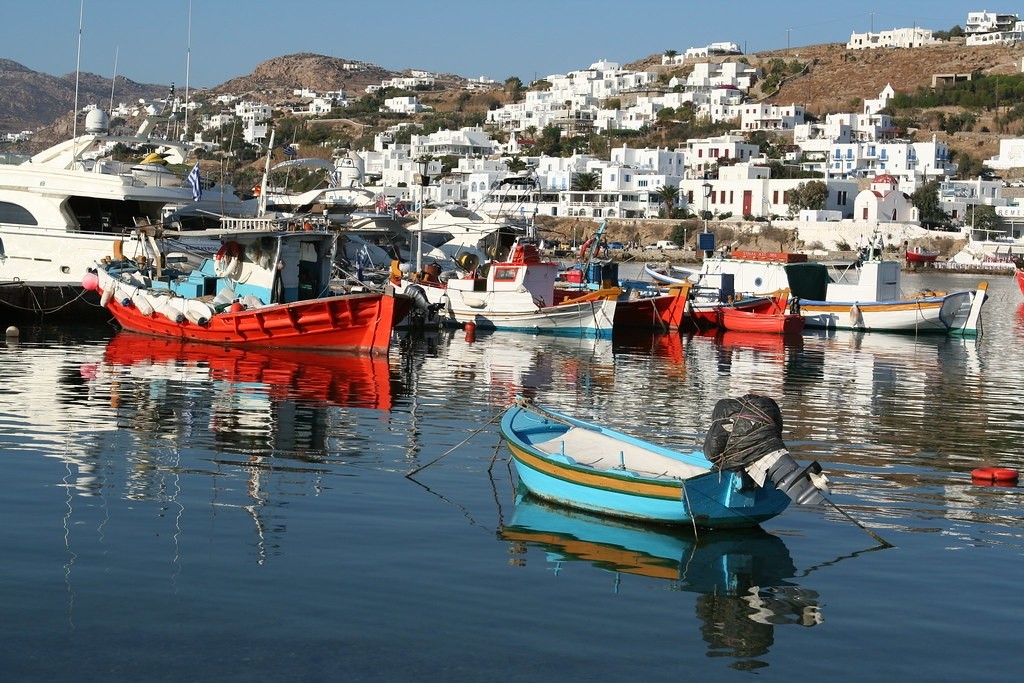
xmin=580 ymin=238 xmax=600 ymax=261
xmin=971 ymin=468 xmax=1020 ymax=482
xmin=213 ymin=241 xmax=240 ymax=278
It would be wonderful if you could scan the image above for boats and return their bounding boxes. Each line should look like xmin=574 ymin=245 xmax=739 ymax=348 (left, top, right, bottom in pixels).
xmin=500 ymin=474 xmax=819 ymax=675
xmin=1013 ymin=255 xmax=1024 ymax=296
xmin=498 ymin=389 xmax=833 ymax=534
xmin=712 ymin=328 xmax=804 ymax=351
xmin=0 ymin=0 xmax=991 ymax=354
xmin=611 ymin=323 xmax=684 ymax=363
xmin=103 ymin=331 xmax=392 ymax=411
xmin=799 ymin=326 xmax=978 ymax=356
xmin=442 ymin=324 xmax=615 ymax=367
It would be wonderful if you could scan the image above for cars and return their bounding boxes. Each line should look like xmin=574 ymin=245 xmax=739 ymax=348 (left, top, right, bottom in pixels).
xmin=645 ymin=243 xmax=657 ymax=250
xmin=608 ymin=241 xmax=624 ymax=249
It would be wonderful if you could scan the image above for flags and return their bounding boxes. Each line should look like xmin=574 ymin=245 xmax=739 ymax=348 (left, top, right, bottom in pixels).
xmin=331 ymin=174 xmax=337 ymax=188
xmin=283 ymin=146 xmax=297 ymax=156
xmin=188 ymin=163 xmax=202 ymax=201
xmin=397 ymin=203 xmax=408 ymax=216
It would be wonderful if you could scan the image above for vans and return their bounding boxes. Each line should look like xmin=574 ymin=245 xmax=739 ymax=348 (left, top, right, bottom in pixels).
xmin=657 ymin=240 xmax=680 ymax=250
